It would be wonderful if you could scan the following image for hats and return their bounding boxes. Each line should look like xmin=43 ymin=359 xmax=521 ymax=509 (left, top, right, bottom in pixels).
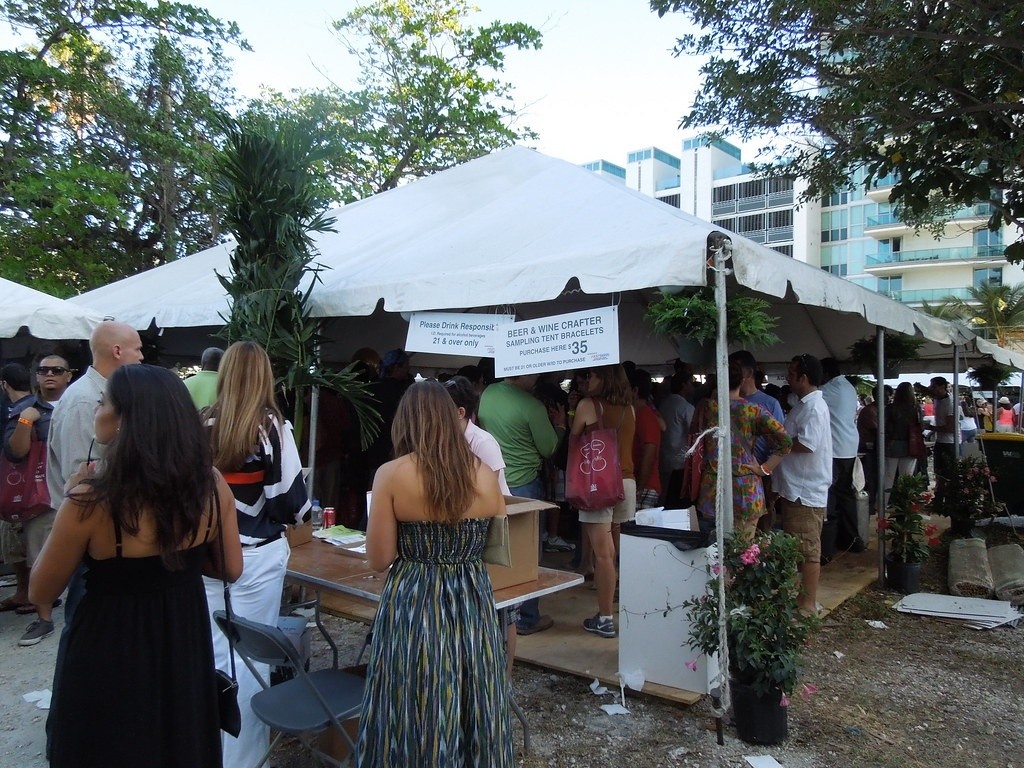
xmin=927 ymin=377 xmax=949 ymax=387
xmin=976 ymin=399 xmax=987 ymax=405
xmin=894 ymin=382 xmax=914 ymax=391
xmin=998 ymin=396 xmax=1010 ymax=404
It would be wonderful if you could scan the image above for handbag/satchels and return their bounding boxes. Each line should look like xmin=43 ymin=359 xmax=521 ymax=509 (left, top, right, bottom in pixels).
xmin=908 ymin=404 xmax=927 ymax=458
xmin=565 ymin=397 xmax=625 ymax=512
xmin=215 ymin=668 xmax=241 ymax=738
xmin=0 ymin=421 xmax=51 ymax=523
xmin=679 ymin=400 xmax=704 ymax=502
xmin=481 ymin=514 xmax=513 ymax=569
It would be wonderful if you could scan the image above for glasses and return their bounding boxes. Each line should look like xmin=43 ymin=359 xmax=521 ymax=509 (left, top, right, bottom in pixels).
xmin=801 ymin=352 xmax=810 ymax=372
xmin=38 ymin=365 xmax=70 ymax=375
xmin=443 ymin=379 xmax=460 ymax=392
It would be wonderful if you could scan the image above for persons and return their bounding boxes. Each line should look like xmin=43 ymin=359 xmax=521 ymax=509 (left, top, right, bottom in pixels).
xmin=0 ymin=319 xmax=1024 ymax=768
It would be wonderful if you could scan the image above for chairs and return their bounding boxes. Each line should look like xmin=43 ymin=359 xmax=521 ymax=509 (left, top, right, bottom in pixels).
xmin=211 ymin=608 xmax=366 ymax=768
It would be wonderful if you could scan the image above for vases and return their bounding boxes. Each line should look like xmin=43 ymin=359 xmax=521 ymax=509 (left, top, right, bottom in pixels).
xmin=726 ymin=676 xmax=792 ymax=748
xmin=950 ymin=513 xmax=975 ymax=539
xmin=886 ymin=554 xmax=921 ymax=594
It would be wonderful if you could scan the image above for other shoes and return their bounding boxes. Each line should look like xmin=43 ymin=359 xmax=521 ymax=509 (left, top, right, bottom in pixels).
xmin=517 ymin=615 xmax=553 ymax=635
xmin=923 ymin=499 xmax=944 ymax=510
xmin=789 ymin=606 xmax=819 ymax=625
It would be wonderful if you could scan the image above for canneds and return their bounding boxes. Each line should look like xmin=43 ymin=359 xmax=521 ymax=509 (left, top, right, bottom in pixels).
xmin=322 ymin=507 xmax=335 ymax=530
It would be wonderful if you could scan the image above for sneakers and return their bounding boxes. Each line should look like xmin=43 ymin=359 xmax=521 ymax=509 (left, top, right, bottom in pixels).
xmin=19 ymin=617 xmax=55 ymax=646
xmin=583 ymin=612 xmax=616 ymax=638
xmin=541 ymin=531 xmax=576 ymax=552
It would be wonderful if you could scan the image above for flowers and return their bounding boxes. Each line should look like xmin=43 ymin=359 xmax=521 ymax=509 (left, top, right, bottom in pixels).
xmin=875 ymin=472 xmax=941 ymax=562
xmin=954 ymin=450 xmax=997 ymax=518
xmin=684 ymin=528 xmax=820 ymax=705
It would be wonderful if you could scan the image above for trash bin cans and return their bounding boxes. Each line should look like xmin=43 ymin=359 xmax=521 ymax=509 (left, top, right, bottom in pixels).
xmin=975 ymin=432 xmax=1024 ymax=517
xmin=619 ymin=519 xmax=726 ymax=695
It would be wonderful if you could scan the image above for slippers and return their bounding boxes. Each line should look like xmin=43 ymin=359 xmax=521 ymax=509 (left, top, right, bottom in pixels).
xmin=1 ymin=597 xmax=26 ymax=611
xmin=15 ymin=598 xmax=62 ymax=614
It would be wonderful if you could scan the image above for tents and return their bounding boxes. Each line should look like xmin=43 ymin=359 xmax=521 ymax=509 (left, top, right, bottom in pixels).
xmin=0 ymin=144 xmax=1024 ymax=709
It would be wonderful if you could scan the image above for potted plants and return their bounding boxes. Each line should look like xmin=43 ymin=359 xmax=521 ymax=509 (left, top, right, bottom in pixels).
xmin=846 ymin=329 xmax=929 ymax=380
xmin=968 ymin=365 xmax=1011 ymax=391
xmin=643 ymin=285 xmax=780 ymax=362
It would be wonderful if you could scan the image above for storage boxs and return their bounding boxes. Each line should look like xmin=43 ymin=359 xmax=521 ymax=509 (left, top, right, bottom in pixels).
xmin=286 ymin=519 xmax=314 ymax=548
xmin=481 ymin=495 xmax=561 ymax=591
xmin=318 ymin=663 xmax=369 ymax=768
xmin=269 ymin=615 xmax=312 ymax=686
xmin=634 ymin=503 xmax=701 ymax=531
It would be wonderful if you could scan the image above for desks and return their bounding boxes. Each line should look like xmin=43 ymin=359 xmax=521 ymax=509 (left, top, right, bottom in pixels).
xmin=279 ymin=525 xmax=584 ymax=751
xmin=617 ymin=517 xmax=726 ymax=695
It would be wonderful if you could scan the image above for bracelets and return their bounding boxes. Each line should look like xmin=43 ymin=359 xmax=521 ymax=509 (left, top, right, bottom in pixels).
xmin=761 ymin=465 xmax=770 ymax=476
xmin=18 ymin=418 xmax=33 ymax=427
xmin=762 ymin=463 xmax=772 ymax=473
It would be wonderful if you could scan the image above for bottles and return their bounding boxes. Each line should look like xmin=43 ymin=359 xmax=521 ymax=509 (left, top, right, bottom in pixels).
xmin=310 ymin=500 xmax=324 ymax=533
